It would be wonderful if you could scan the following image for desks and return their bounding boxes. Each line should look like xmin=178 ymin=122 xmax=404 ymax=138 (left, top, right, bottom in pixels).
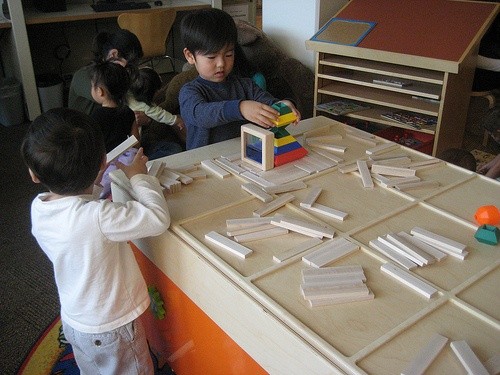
xmin=108 ymin=115 xmax=500 ymax=375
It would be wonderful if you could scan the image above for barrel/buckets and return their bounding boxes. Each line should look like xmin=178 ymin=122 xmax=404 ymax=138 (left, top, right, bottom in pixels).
xmin=36 ymin=73 xmax=64 ymax=113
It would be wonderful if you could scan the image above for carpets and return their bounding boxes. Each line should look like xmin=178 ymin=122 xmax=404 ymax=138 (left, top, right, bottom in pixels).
xmin=14 ymin=312 xmax=184 ymax=375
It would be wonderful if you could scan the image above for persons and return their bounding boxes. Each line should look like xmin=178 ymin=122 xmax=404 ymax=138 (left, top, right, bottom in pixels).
xmin=20 ymin=106 xmax=171 ymax=375
xmin=178 ymin=8 xmax=301 ymax=151
xmin=66 ymin=28 xmax=184 ymax=160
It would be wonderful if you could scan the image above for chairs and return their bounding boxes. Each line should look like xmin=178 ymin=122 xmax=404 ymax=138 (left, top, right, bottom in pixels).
xmin=117 ymin=9 xmax=177 ymax=81
xmin=165 ymin=20 xmax=318 ymax=149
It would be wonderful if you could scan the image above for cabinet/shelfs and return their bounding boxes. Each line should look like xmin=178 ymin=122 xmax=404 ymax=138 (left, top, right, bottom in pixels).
xmin=306 ymin=0 xmax=500 ymax=157
xmin=0 ymin=0 xmax=260 ymax=122
